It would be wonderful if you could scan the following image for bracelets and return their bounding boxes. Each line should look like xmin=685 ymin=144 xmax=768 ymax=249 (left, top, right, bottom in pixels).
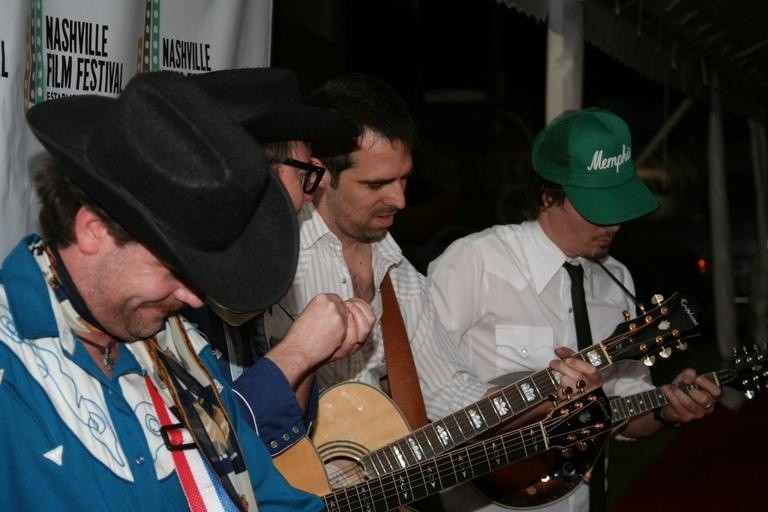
xmin=653 ymin=407 xmax=667 ymax=426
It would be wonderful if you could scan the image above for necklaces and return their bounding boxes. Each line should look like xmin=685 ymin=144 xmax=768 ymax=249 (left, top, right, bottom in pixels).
xmin=72 ymin=329 xmax=116 ymax=372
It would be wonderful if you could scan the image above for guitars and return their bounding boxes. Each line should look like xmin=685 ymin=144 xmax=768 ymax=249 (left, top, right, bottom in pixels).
xmin=311 ymin=294 xmax=702 ymax=491
xmin=273 ymin=380 xmax=614 ymax=511
xmin=470 ymin=345 xmax=766 ymax=511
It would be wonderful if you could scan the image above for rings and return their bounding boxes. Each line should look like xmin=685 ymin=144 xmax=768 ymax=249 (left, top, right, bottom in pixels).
xmin=705 ymin=400 xmax=713 ymax=410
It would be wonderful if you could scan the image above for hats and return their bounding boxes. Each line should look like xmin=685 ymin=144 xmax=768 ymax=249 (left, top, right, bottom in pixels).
xmin=189 ymin=68 xmax=361 ymax=156
xmin=531 ymin=107 xmax=660 ymax=224
xmin=27 ymin=70 xmax=301 ymax=316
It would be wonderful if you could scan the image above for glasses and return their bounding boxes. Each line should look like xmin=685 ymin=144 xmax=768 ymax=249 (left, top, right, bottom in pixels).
xmin=267 ymin=158 xmax=325 ymax=194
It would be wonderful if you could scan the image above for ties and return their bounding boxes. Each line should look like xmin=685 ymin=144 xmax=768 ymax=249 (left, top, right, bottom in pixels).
xmin=561 ymin=262 xmax=606 ymax=512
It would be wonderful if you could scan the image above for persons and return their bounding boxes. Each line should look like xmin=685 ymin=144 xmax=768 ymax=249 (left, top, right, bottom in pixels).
xmin=0 ymin=71 xmax=325 ymax=512
xmin=259 ymin=73 xmax=600 ymax=511
xmin=186 ymin=84 xmax=374 ymax=456
xmin=428 ymin=108 xmax=722 ymax=512
